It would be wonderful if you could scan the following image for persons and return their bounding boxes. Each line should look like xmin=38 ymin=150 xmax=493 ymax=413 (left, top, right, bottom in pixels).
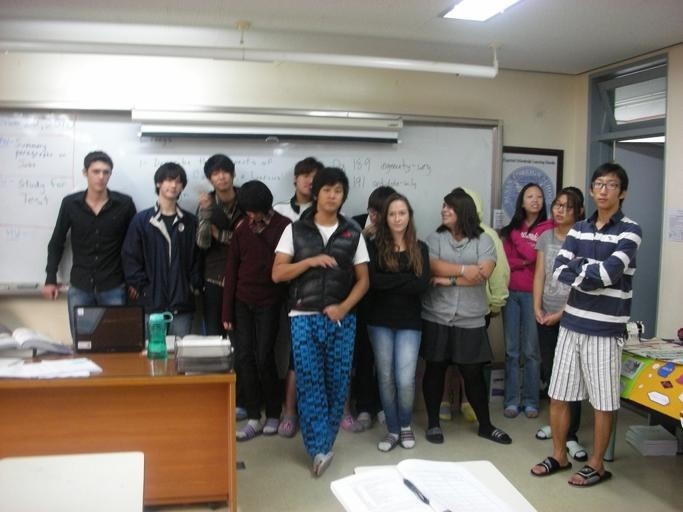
xmin=531 ymin=162 xmax=643 ymax=487
xmin=352 ymin=184 xmax=588 ymax=452
xmin=121 ymin=162 xmax=205 ymax=340
xmin=42 ymin=151 xmax=137 ymax=345
xmin=198 ymin=155 xmax=370 ymax=476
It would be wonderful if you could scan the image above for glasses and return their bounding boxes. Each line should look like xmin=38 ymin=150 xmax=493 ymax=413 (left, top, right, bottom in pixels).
xmin=591 ymin=177 xmax=621 ymax=191
xmin=552 ymin=199 xmax=574 ymax=213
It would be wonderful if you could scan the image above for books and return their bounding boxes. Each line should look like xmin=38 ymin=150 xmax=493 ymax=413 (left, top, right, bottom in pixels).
xmin=0 ymin=328 xmax=72 ymax=358
xmin=624 ymin=424 xmax=678 ymax=457
xmin=330 ymin=459 xmax=539 ymax=512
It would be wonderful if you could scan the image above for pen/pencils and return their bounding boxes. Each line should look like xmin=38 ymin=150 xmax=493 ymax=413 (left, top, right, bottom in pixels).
xmin=403 ymin=478 xmax=430 ymax=504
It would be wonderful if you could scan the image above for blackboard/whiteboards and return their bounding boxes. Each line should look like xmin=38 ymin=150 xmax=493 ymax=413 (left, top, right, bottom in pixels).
xmin=0 ymin=99 xmax=503 ymax=297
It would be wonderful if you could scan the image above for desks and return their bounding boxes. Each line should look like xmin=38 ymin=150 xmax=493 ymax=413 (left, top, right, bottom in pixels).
xmin=602 ymin=336 xmax=683 ymax=462
xmin=0 ymin=350 xmax=237 ymax=512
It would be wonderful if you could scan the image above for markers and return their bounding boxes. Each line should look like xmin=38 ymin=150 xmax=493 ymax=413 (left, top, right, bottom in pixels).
xmin=16 ymin=283 xmax=38 ymax=288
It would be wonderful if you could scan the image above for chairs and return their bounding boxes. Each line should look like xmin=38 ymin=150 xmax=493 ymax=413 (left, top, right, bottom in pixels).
xmin=72 ymin=303 xmax=146 ymax=354
xmin=1 ymin=451 xmax=145 ymax=512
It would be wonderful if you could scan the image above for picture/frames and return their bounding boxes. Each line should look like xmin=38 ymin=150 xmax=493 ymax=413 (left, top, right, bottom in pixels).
xmin=501 ymin=145 xmax=565 ymax=237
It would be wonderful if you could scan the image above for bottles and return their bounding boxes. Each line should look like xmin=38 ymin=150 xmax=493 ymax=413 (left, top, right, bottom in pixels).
xmin=147 ymin=310 xmax=173 ymax=360
